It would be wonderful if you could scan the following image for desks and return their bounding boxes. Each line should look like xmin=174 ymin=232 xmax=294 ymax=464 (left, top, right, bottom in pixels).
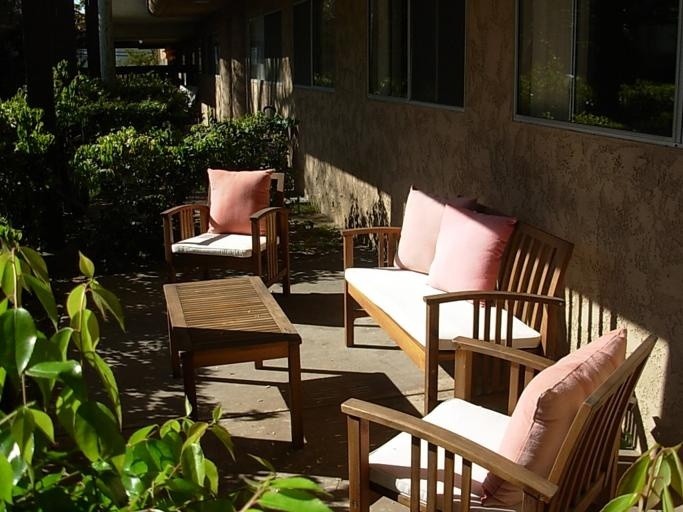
xmin=157 ymin=275 xmax=304 ymax=454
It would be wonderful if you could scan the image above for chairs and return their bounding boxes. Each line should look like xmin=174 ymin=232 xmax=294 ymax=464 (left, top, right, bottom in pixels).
xmin=334 ymin=334 xmax=661 ymax=512
xmin=161 ymin=172 xmax=294 ymax=307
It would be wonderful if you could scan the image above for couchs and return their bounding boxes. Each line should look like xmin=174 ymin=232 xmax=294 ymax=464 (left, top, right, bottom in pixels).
xmin=339 ymin=194 xmax=575 ymax=419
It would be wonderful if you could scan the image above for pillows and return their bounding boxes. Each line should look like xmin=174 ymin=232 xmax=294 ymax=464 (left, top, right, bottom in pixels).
xmin=207 ymin=168 xmax=277 ymax=236
xmin=479 ymin=329 xmax=628 ymax=509
xmin=393 ymin=185 xmax=520 ymax=308
